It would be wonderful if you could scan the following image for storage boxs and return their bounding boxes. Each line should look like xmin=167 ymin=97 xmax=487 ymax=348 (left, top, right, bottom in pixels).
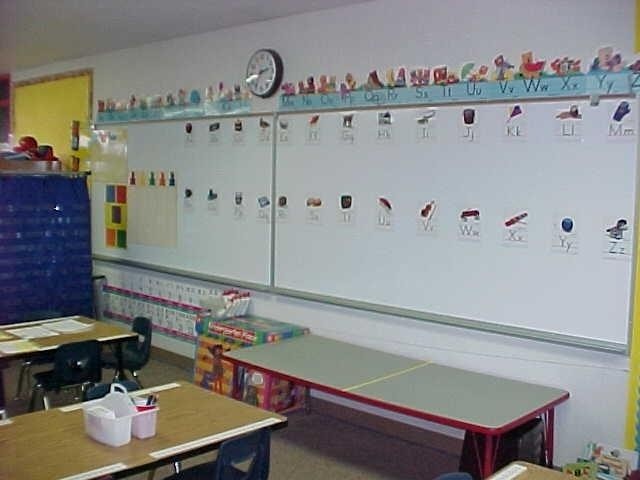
xmin=192 ymin=314 xmax=309 ymax=416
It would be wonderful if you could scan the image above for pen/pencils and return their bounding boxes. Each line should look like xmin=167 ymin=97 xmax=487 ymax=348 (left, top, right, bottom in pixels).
xmin=146 ymin=393 xmax=159 ymax=406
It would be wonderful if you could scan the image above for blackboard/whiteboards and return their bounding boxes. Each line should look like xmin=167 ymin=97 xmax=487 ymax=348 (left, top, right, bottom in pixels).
xmin=89 ymin=68 xmax=639 ymax=359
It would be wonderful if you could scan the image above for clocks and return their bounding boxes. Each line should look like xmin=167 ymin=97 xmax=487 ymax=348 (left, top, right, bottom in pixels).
xmin=247 ymin=48 xmax=284 ymax=98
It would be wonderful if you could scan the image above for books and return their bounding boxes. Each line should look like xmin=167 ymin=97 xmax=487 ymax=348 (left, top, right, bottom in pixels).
xmin=562 ymin=440 xmax=639 ymax=480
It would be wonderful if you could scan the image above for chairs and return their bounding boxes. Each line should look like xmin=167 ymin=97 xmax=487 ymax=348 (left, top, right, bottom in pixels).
xmin=13 ymin=340 xmax=102 ymax=413
xmin=83 ymin=381 xmax=147 ymax=399
xmin=163 ymin=431 xmax=271 ymax=480
xmin=101 ymin=316 xmax=152 ymax=388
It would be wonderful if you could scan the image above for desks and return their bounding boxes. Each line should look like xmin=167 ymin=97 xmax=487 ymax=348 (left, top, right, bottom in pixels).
xmin=1 ymin=378 xmax=289 ymax=480
xmin=0 ymin=314 xmax=139 ymax=382
xmin=220 ymin=333 xmax=570 ymax=479
